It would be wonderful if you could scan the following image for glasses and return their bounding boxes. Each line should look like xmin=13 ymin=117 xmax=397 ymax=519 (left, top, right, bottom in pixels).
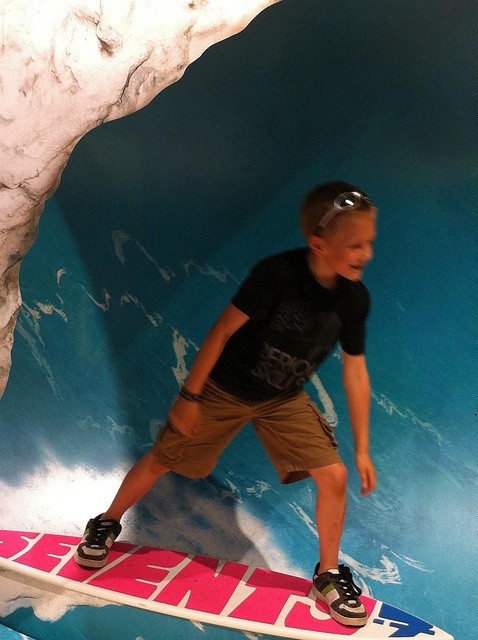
xmin=318 ymin=189 xmax=371 ymax=229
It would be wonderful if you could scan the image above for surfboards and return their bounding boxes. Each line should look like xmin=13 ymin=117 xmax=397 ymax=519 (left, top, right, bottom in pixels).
xmin=0 ymin=530 xmax=454 ymax=640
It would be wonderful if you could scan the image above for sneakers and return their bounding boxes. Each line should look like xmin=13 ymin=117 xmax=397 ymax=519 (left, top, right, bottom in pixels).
xmin=76 ymin=513 xmax=121 ymax=570
xmin=311 ymin=562 xmax=367 ymax=627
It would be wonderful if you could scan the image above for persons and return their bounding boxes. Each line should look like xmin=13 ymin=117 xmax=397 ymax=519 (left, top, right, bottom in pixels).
xmin=72 ymin=179 xmax=377 ymax=628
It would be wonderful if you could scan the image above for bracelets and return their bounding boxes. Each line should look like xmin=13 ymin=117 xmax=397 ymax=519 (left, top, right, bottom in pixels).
xmin=179 ymin=387 xmax=204 ymax=403
